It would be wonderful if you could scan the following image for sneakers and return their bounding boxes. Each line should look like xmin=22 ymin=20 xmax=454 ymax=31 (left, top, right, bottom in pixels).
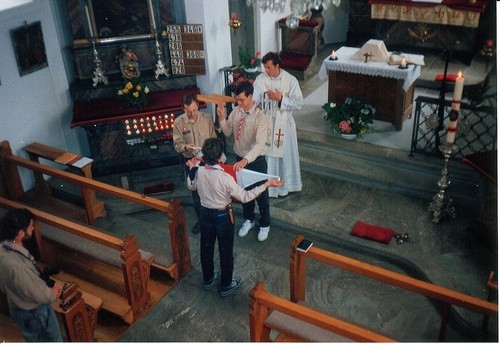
xmin=238 ymin=218 xmax=254 ymax=236
xmin=258 ymin=226 xmax=270 ymax=241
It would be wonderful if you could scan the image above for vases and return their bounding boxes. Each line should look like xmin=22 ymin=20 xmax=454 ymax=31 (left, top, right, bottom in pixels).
xmin=340 ymin=134 xmax=356 ymax=140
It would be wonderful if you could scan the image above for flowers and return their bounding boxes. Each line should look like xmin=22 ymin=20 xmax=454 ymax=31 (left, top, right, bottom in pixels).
xmin=116 ymin=81 xmax=150 ymax=110
xmin=232 ymin=45 xmax=262 ymax=83
xmin=322 ymin=97 xmax=376 ymax=142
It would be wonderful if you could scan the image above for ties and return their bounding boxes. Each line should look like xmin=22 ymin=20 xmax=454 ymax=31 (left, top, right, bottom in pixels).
xmin=236 ymin=112 xmax=246 ymax=140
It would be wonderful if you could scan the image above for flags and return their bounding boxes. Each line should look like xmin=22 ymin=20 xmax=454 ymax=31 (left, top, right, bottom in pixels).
xmin=197 ymin=157 xmax=281 ymax=190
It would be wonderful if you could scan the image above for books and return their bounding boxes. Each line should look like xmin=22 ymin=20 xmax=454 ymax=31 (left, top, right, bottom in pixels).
xmin=296 ymin=239 xmax=314 ymax=253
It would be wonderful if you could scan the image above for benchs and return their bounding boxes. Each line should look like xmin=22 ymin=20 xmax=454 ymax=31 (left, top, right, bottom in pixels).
xmin=0 ymin=138 xmax=498 ymax=342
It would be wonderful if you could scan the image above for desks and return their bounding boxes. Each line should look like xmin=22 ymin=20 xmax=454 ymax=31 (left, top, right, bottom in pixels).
xmin=319 ymin=46 xmax=420 ymax=131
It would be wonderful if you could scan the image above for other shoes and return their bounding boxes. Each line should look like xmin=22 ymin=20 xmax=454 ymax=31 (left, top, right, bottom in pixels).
xmin=192 ymin=221 xmax=200 ymax=234
xmin=203 ymin=270 xmax=219 ymax=289
xmin=221 ymin=279 xmax=241 ymax=297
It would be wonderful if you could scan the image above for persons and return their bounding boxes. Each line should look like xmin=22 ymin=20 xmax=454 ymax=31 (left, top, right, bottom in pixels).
xmin=252 ymin=52 xmax=304 ymax=197
xmin=187 ymin=138 xmax=283 ymax=295
xmin=172 ymin=95 xmax=218 ymax=233
xmin=217 ymin=81 xmax=271 ymax=240
xmin=0 ymin=207 xmax=66 ymax=342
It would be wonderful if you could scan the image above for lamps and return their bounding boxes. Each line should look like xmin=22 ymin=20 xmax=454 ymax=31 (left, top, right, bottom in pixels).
xmin=229 ymin=12 xmax=243 ymax=31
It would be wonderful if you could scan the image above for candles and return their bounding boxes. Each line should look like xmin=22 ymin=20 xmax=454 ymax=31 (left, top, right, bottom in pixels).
xmin=401 ymin=58 xmax=406 ymax=67
xmin=148 ymin=0 xmax=156 ymax=28
xmin=125 ymin=113 xmax=174 ymax=136
xmin=447 ymin=70 xmax=463 ymax=143
xmin=332 ymin=49 xmax=336 ymax=58
xmin=85 ymin=5 xmax=93 ymax=37
